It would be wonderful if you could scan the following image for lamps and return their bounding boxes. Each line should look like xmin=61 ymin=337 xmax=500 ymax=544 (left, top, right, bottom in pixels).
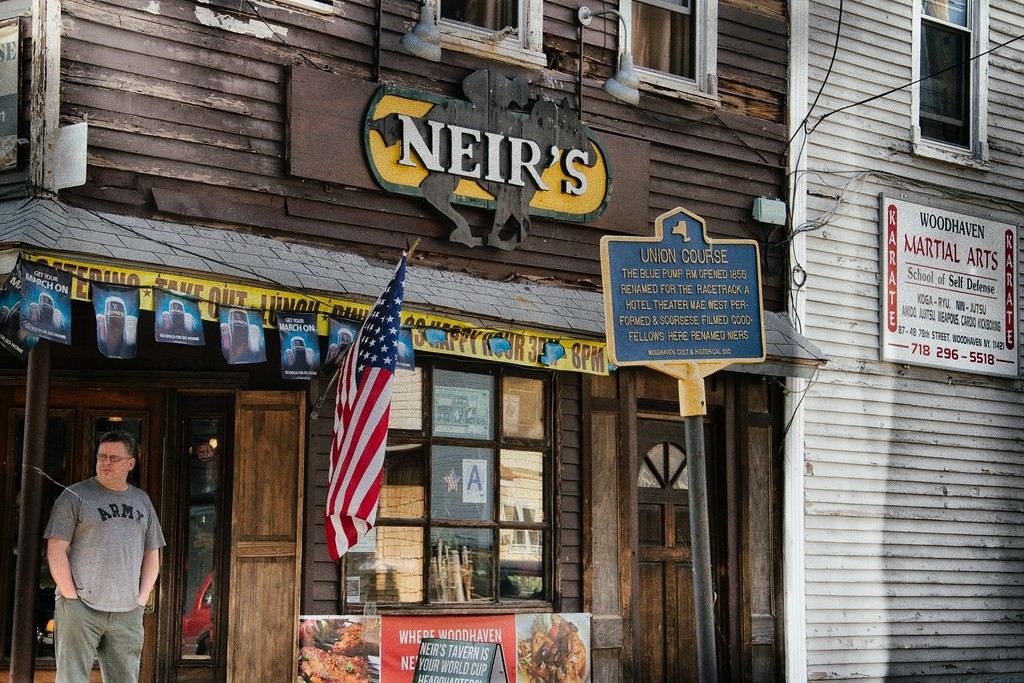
xmin=398 ymin=0 xmax=442 ymax=62
xmin=576 ymin=4 xmax=640 ymax=106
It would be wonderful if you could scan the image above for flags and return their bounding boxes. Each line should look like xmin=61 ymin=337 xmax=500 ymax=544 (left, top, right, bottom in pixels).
xmin=323 ymin=252 xmax=409 ymax=562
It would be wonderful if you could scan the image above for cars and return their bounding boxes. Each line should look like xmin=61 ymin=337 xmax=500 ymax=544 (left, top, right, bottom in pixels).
xmin=36 ymin=603 xmax=56 ymax=657
xmin=182 ymin=572 xmax=213 ymax=661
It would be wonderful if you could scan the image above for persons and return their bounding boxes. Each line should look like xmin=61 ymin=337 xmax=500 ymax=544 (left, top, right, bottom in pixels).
xmin=43 ymin=430 xmax=167 ymax=683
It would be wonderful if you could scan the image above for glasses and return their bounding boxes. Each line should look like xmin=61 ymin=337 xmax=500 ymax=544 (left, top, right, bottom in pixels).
xmin=97 ymin=454 xmax=133 ymax=462
